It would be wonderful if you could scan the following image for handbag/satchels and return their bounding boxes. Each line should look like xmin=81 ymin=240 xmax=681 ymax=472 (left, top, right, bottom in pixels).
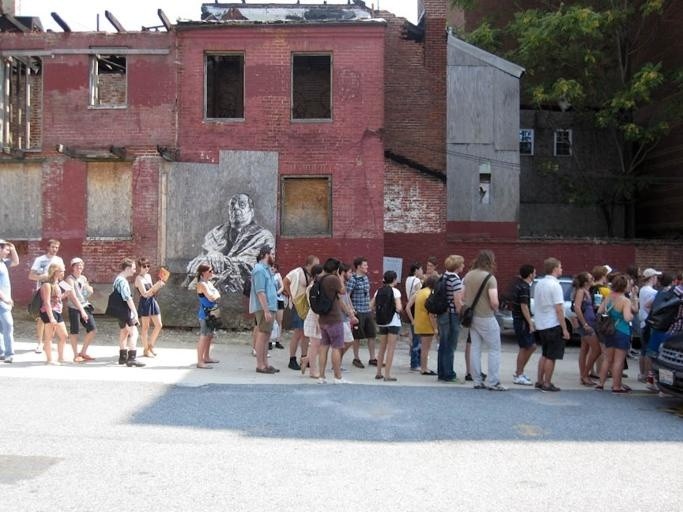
xmin=460 ymin=304 xmax=474 ymax=327
xmin=205 ymin=307 xmax=223 ymax=327
xmin=106 ymin=289 xmax=130 ymax=321
xmin=572 ymin=300 xmax=586 ymax=312
xmin=28 ymin=292 xmax=42 ymax=313
xmin=294 ymin=292 xmax=309 ymax=320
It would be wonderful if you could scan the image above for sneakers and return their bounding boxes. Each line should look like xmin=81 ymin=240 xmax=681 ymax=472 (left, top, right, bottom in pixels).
xmin=440 ymin=370 xmax=504 ymax=393
xmin=34 ymin=346 xmax=97 ymax=366
xmin=511 ymin=373 xmax=563 ymax=392
xmin=579 ymin=373 xmax=647 ymax=392
xmin=197 ymin=357 xmax=220 ymax=370
xmin=0 ymin=354 xmax=13 ymax=362
xmin=253 ymin=341 xmax=437 ymax=384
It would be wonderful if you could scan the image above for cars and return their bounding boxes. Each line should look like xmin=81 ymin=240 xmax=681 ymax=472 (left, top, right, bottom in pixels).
xmin=499 ymin=275 xmax=627 ymax=346
xmin=651 ymin=334 xmax=682 ymax=400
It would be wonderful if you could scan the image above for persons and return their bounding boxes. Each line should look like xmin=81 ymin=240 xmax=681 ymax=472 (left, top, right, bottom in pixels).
xmin=461 ymin=249 xmax=509 ymax=392
xmin=179 ymin=192 xmax=275 ymax=293
xmin=28 ymin=239 xmax=97 ymax=365
xmin=405 ymin=255 xmax=443 ymax=376
xmin=572 ymin=263 xmax=682 ymax=392
xmin=535 ymin=257 xmax=570 ymax=392
xmin=301 ymin=258 xmax=360 ymax=381
xmin=511 ymin=264 xmax=537 ymax=386
xmin=112 ymin=258 xmax=145 ymax=367
xmin=196 ymin=264 xmax=221 ymax=369
xmin=437 ymin=255 xmax=467 ymax=382
xmin=0 ymin=239 xmax=20 ymax=363
xmin=250 ymin=245 xmax=284 ymax=374
xmin=282 ymin=255 xmax=320 ymax=370
xmin=135 ymin=256 xmax=165 ymax=358
xmin=369 ymin=270 xmax=403 ymax=381
xmin=347 ymin=257 xmax=385 ymax=368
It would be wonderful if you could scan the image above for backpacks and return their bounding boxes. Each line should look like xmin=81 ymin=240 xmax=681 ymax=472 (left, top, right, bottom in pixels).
xmin=310 ymin=278 xmax=332 ymax=314
xmin=598 ymin=313 xmax=615 ymax=336
xmin=375 ymin=287 xmax=395 ymax=324
xmin=425 ymin=275 xmax=450 ymax=314
xmin=645 ymin=289 xmax=682 ymax=332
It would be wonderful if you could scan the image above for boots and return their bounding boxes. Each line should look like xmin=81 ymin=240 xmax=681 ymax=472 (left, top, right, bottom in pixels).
xmin=120 ymin=348 xmax=158 ymax=366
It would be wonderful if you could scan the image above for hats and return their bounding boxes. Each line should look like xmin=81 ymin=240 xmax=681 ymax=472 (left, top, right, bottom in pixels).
xmin=71 ymin=258 xmax=83 ymax=266
xmin=643 ymin=268 xmax=662 ymax=278
